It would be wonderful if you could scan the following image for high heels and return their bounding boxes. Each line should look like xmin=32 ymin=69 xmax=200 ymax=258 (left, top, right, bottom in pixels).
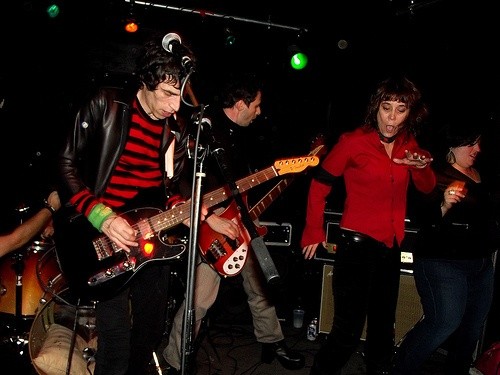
xmin=157 ymin=353 xmax=178 ymax=375
xmin=259 ymin=340 xmax=304 ymax=371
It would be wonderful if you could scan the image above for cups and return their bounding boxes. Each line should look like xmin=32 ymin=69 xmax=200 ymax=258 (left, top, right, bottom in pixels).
xmin=293 ymin=309 xmax=304 ymax=328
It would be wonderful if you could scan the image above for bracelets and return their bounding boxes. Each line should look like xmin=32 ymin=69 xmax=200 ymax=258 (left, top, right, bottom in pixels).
xmin=440 ymin=201 xmax=449 ymax=210
xmin=44 ymin=204 xmax=57 ymax=214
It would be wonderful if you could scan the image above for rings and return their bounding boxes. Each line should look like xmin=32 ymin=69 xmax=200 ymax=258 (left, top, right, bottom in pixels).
xmin=449 ymin=190 xmax=454 ymax=195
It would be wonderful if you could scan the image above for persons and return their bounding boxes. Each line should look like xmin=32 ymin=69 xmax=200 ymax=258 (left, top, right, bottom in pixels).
xmin=392 ymin=122 xmax=500 ymax=375
xmin=56 ymin=36 xmax=209 ymax=375
xmin=299 ymin=77 xmax=437 ymax=375
xmin=161 ymin=74 xmax=304 ymax=375
xmin=0 ymin=189 xmax=60 ymax=257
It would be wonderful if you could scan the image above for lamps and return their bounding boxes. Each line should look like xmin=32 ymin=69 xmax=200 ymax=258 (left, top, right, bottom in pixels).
xmin=287 ymin=34 xmax=308 ymax=70
xmin=124 ymin=4 xmax=138 ymax=33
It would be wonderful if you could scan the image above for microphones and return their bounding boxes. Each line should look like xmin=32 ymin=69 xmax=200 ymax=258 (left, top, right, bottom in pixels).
xmin=162 ymin=33 xmax=195 ymax=71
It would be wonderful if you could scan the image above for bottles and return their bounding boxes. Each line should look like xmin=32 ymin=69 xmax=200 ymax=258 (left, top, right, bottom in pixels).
xmin=307 ymin=318 xmax=318 ymax=341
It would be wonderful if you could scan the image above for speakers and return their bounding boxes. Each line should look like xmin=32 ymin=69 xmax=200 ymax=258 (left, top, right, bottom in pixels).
xmin=319 ymin=263 xmax=425 ymax=346
